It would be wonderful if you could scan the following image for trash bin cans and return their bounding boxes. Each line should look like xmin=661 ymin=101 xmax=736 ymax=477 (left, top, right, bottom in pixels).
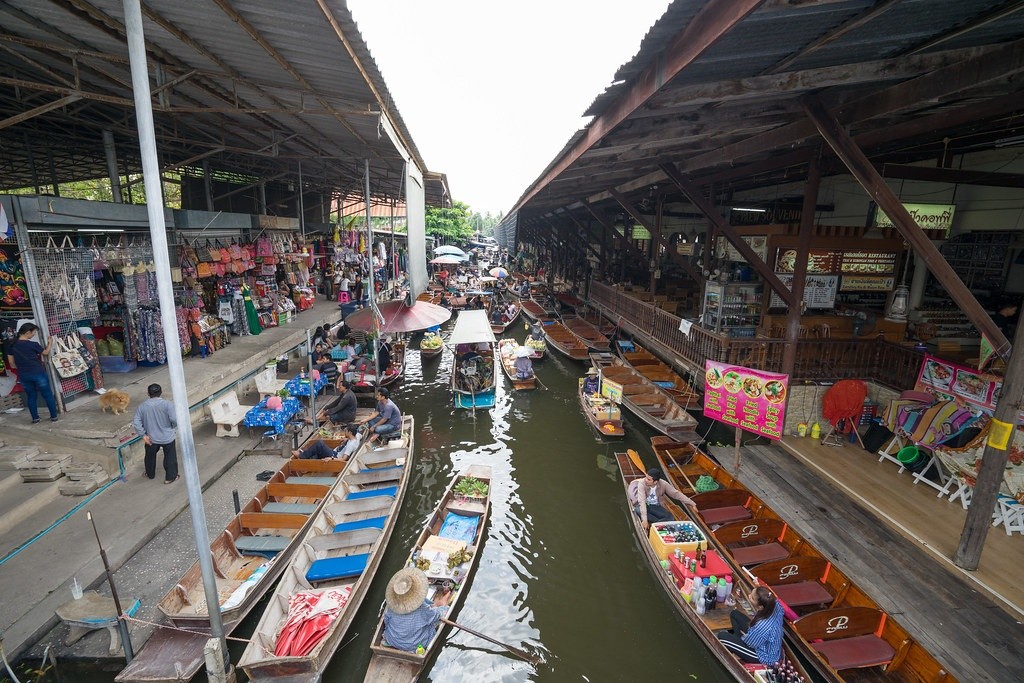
xmin=277 ymin=358 xmax=288 ymax=374
xmin=338 ymin=303 xmax=360 ymax=321
xmin=362 ymin=294 xmax=369 ymax=308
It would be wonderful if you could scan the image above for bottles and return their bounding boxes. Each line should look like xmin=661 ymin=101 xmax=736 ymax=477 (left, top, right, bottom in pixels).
xmin=300 ymin=367 xmax=304 ymax=380
xmin=798 ymin=423 xmax=807 ymax=437
xmin=811 ymin=422 xmax=821 ymax=439
xmin=666 ymin=524 xmax=704 ymax=542
xmin=692 ymin=576 xmax=733 ymax=615
xmin=695 ymin=542 xmax=702 ymax=560
xmin=700 ymin=550 xmax=706 ymax=568
xmin=275 ymin=390 xmax=279 ymax=397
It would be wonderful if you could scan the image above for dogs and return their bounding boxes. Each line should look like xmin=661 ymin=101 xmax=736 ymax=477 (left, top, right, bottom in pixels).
xmin=97 ymin=388 xmax=129 ymax=415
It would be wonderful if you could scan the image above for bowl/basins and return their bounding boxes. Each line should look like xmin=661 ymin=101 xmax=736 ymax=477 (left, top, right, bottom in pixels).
xmin=705 ymin=368 xmax=786 ymax=405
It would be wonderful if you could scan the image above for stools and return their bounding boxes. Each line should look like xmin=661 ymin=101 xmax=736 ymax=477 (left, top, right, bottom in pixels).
xmin=288 ymin=418 xmax=309 ymax=437
xmin=261 ymin=431 xmax=284 ymax=449
xmin=738 ymin=658 xmax=770 ymax=673
xmin=338 ymin=292 xmax=348 ymax=303
xmin=53 ymin=589 xmax=140 ymax=656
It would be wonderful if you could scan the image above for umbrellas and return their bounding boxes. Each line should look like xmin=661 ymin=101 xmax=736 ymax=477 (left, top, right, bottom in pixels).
xmin=488 ymin=266 xmax=509 ymax=277
xmin=428 ymin=244 xmax=466 ymax=264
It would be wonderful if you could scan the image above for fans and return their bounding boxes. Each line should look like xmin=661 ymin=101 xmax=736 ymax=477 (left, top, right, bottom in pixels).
xmin=354 ymin=358 xmax=373 ymax=387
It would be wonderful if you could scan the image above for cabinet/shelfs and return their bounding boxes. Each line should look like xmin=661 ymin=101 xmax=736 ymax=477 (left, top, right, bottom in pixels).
xmin=700 ymin=280 xmax=763 ymax=336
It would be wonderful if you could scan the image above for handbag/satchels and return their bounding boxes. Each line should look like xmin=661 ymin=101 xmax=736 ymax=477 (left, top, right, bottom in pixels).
xmin=51 ymin=336 xmax=89 ymax=378
xmin=178 ymin=231 xmax=309 ymax=323
xmin=67 ymin=332 xmax=95 ymax=368
xmin=40 ymin=234 xmax=152 ymax=316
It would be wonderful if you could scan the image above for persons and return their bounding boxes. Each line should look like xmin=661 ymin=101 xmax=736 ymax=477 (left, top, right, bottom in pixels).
xmin=311 ymin=323 xmax=338 ymax=353
xmin=441 ymin=279 xmax=528 ymax=325
xmin=7 ymin=322 xmax=58 ymax=423
xmin=634 ymin=468 xmax=699 ymax=531
xmin=346 ymin=338 xmax=360 ymax=366
xmin=322 ymin=264 xmax=364 ymax=310
xmin=514 ymin=346 xmax=535 ymax=379
xmin=291 ymin=422 xmax=363 ymax=464
xmin=455 ymin=344 xmax=480 ymax=363
xmin=358 ymin=387 xmax=401 ymax=443
xmin=527 ymin=321 xmax=546 ymax=340
xmin=276 ymin=278 xmax=290 ymax=297
xmin=378 ymin=334 xmax=392 ymax=375
xmin=382 ymin=567 xmax=441 ymax=652
xmin=718 ymin=586 xmax=785 ymax=666
xmin=319 ymin=353 xmax=338 ymax=383
xmin=316 ymin=380 xmax=357 ymax=422
xmin=307 ymin=343 xmax=323 ymax=372
xmin=133 ymin=383 xmax=180 ymax=485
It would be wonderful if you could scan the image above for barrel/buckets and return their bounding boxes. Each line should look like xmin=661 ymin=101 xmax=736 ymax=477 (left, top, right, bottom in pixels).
xmin=278 ymin=358 xmax=289 ymax=373
xmin=903 ymin=450 xmax=938 ymax=481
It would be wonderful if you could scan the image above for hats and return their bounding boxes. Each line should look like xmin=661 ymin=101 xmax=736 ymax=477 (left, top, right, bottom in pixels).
xmin=584 ymin=367 xmax=598 ymax=374
xmin=385 ymin=568 xmax=428 ymax=614
xmin=514 ymin=345 xmax=534 ymax=357
xmin=380 ymin=335 xmax=387 ymax=339
xmin=533 ymin=321 xmax=541 ymax=326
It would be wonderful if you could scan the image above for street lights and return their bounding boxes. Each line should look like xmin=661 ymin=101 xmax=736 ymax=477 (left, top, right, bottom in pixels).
xmin=476 ymin=215 xmax=478 ymax=242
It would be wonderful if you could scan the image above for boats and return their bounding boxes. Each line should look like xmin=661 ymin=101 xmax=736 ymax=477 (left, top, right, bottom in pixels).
xmin=415 ymin=247 xmax=585 ymax=334
xmin=588 ymin=352 xmax=706 ymax=445
xmin=362 ymin=464 xmax=494 ymax=683
xmin=538 ymin=317 xmax=591 ymax=360
xmin=561 ymin=314 xmax=612 ymax=352
xmin=613 ymin=448 xmax=814 ymax=683
xmin=497 ymin=338 xmax=537 ymax=390
xmin=234 ymin=411 xmax=417 ymax=683
xmin=578 ymin=377 xmax=626 ymax=437
xmin=448 ymin=309 xmax=499 ymax=410
xmin=114 ymin=407 xmax=379 ymax=683
xmin=524 ymin=335 xmax=547 ymax=359
xmin=650 ymin=435 xmax=958 ymax=683
xmin=575 ymin=306 xmax=617 ymax=335
xmin=615 ymin=339 xmax=704 ymax=410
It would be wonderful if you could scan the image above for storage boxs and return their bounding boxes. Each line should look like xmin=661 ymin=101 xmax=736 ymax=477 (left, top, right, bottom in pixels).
xmin=275 ymin=311 xmax=292 ymax=327
xmin=754 ymin=669 xmax=779 ymax=683
xmin=596 ymin=407 xmax=621 ymax=421
xmin=293 ymin=293 xmax=306 ymax=304
xmin=98 ymin=355 xmax=136 ymax=373
xmin=649 ymin=520 xmax=708 ymax=561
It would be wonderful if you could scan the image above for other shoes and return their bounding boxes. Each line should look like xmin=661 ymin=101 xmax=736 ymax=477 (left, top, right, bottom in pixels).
xmin=51 ymin=415 xmax=58 ymax=421
xmin=144 ymin=471 xmax=155 ymax=480
xmin=34 ymin=417 xmax=42 ymax=423
xmin=164 ymin=475 xmax=179 ymax=483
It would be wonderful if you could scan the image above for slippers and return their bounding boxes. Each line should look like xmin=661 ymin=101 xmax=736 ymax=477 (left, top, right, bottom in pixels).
xmin=256 ymin=471 xmax=274 ymax=480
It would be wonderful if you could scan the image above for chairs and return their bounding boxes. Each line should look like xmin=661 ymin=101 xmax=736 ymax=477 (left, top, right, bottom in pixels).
xmin=873 ymin=418 xmax=1024 ymax=536
xmin=608 ymin=277 xmax=702 ymax=318
xmin=323 ymin=367 xmax=337 ymax=396
xmin=768 ymin=322 xmax=831 ymax=362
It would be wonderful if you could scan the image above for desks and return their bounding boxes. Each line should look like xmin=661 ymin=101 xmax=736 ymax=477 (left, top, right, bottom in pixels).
xmin=242 ymin=396 xmax=300 ymax=441
xmin=331 ymin=340 xmax=362 ymax=362
xmin=668 ymin=549 xmax=733 ymax=592
xmin=285 ymin=372 xmax=328 ymax=407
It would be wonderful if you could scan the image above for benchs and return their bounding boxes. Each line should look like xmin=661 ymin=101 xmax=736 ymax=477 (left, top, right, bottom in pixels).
xmin=601 ymin=350 xmax=697 ymax=416
xmin=731 ymin=539 xmax=790 ymax=565
xmin=209 ymin=389 xmax=255 ymax=438
xmin=358 ymin=463 xmax=405 ymax=474
xmin=811 ymin=634 xmax=897 ymax=674
xmin=285 ymin=476 xmax=336 ymax=486
xmin=344 ymin=485 xmax=399 ymax=500
xmin=232 ymin=535 xmax=292 ymax=559
xmin=251 ymin=365 xmax=290 ymax=403
xmin=306 ymin=552 xmax=371 ymax=589
xmin=262 ymin=502 xmax=318 ymax=516
xmin=700 ymin=503 xmax=753 ymax=525
xmin=445 ymin=499 xmax=488 ymax=517
xmin=332 ymin=516 xmax=388 ymax=534
xmin=769 ymin=579 xmax=835 ymax=607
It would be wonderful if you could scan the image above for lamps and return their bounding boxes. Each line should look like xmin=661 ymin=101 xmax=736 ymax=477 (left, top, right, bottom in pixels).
xmin=883 ymin=243 xmax=921 ymax=324
xmin=862 ymin=164 xmax=886 ymax=242
